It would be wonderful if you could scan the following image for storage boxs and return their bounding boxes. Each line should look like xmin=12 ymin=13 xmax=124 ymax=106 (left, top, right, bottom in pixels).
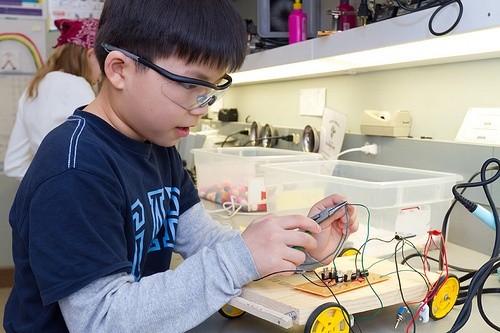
xmin=189 ymin=146 xmax=323 ymax=212
xmin=259 ymin=158 xmax=463 ymax=258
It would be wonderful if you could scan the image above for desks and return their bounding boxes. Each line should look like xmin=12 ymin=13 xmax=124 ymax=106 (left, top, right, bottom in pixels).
xmin=171 ymin=199 xmax=500 ymax=333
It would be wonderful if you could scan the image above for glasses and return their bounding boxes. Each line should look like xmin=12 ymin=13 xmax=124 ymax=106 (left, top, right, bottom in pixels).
xmin=102 ymin=43 xmax=233 ymax=111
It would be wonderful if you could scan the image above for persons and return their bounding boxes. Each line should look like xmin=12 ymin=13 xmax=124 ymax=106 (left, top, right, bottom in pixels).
xmin=3 ymin=0 xmax=360 ymax=333
xmin=2 ymin=17 xmax=102 ymax=182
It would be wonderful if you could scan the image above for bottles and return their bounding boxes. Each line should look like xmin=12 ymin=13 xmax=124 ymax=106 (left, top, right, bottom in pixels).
xmin=338 ymin=0 xmax=356 ymax=31
xmin=287 ymin=0 xmax=307 ymax=45
xmin=356 ymin=0 xmax=373 ymax=29
